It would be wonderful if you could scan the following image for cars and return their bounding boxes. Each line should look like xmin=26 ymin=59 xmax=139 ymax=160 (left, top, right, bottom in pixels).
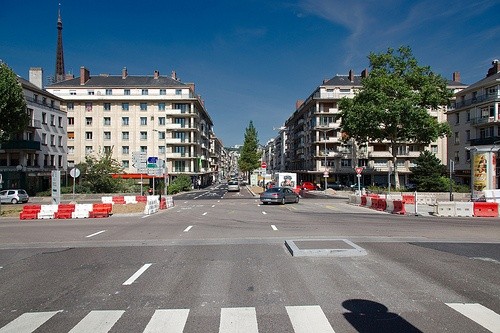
xmin=350 ymin=183 xmax=366 ymax=190
xmin=327 ymin=180 xmax=348 ymax=189
xmin=260 ymin=188 xmax=299 ymax=205
xmin=227 ymin=180 xmax=240 ymax=192
xmin=297 ymin=182 xmax=320 ymax=191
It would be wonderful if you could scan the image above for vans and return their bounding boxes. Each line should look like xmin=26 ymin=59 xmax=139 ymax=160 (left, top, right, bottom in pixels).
xmin=0 ymin=189 xmax=29 ymax=205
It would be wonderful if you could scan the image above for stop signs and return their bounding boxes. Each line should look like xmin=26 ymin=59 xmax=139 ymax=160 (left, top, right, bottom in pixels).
xmin=261 ymin=162 xmax=266 ymax=168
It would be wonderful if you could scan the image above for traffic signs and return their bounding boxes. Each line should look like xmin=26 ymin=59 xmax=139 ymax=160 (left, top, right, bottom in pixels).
xmin=132 ymin=152 xmax=148 ymax=173
